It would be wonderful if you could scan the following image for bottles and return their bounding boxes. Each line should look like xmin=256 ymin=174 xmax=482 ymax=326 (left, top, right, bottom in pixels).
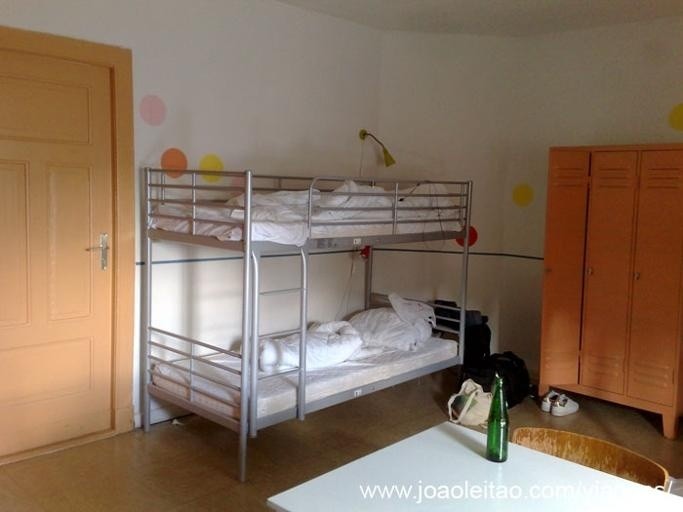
xmin=484 ymin=378 xmax=508 ymax=461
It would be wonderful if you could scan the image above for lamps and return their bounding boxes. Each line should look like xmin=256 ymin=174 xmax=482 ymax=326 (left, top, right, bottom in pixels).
xmin=358 ymin=127 xmax=396 ymax=168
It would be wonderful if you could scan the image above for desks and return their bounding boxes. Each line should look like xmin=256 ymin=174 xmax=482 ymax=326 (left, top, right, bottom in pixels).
xmin=264 ymin=419 xmax=682 ymax=512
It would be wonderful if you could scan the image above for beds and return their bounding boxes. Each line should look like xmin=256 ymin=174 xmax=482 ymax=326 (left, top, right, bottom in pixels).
xmin=134 ymin=164 xmax=479 ymax=484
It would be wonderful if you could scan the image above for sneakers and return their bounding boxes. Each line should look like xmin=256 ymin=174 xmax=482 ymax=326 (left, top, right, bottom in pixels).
xmin=551 ymin=394 xmax=580 ymax=416
xmin=541 ymin=390 xmax=559 ymax=412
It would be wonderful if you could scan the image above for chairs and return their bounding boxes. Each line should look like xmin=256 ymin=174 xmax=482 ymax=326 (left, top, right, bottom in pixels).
xmin=509 ymin=422 xmax=669 ymax=495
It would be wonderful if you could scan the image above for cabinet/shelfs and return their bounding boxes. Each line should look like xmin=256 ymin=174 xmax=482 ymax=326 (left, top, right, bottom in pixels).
xmin=531 ymin=141 xmax=682 ymax=443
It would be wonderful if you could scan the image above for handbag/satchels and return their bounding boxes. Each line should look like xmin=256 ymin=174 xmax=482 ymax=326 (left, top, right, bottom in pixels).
xmin=432 ymin=299 xmax=530 ymax=426
